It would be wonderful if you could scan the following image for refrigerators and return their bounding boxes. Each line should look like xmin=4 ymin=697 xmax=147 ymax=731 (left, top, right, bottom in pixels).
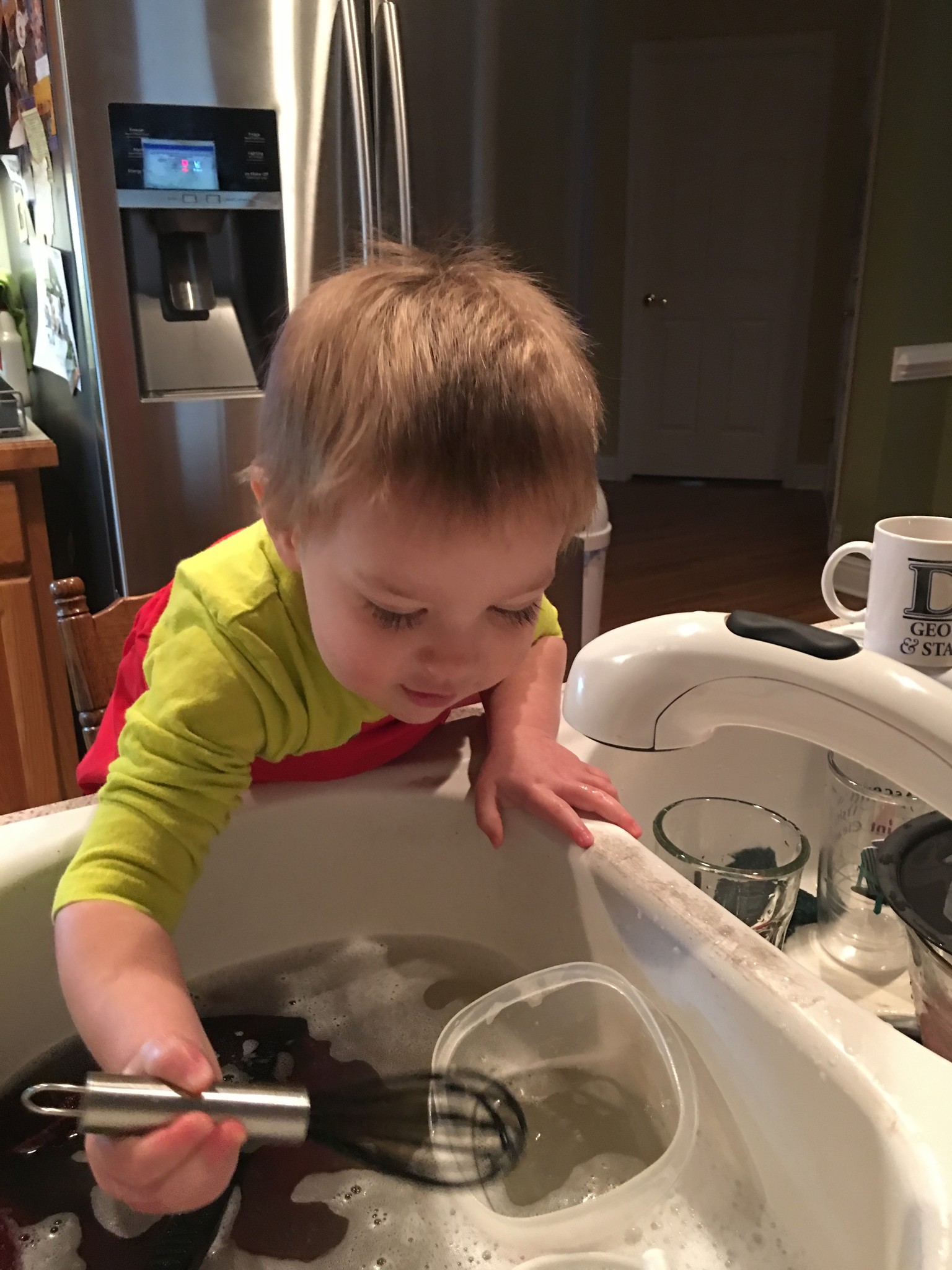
xmin=3 ymin=0 xmax=590 ymax=615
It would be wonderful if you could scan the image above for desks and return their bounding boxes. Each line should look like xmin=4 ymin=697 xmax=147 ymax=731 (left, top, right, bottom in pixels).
xmin=0 ymin=412 xmax=89 ymax=812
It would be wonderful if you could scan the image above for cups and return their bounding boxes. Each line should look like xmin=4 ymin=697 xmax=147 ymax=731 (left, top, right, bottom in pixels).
xmin=853 ymin=812 xmax=951 ymax=1063
xmin=653 ymin=797 xmax=812 ymax=956
xmin=820 ymin=748 xmax=940 ymax=973
xmin=821 ymin=515 xmax=951 ymax=670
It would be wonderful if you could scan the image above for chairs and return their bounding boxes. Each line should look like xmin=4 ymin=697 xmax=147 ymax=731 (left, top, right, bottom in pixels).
xmin=49 ymin=575 xmax=159 ymax=745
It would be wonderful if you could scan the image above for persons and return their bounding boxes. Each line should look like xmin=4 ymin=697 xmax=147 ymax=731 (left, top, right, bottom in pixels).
xmin=49 ymin=229 xmax=641 ymax=1216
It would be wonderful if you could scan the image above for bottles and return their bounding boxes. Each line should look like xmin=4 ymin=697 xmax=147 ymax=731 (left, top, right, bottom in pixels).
xmin=0 ymin=300 xmax=32 ymax=407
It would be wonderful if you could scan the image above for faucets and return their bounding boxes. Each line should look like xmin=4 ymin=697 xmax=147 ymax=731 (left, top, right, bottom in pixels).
xmin=556 ymin=604 xmax=952 ymax=816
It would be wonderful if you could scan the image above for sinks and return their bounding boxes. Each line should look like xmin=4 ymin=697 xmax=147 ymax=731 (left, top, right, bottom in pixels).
xmin=510 ymin=679 xmax=952 ymax=1138
xmin=0 ymin=763 xmax=952 ymax=1270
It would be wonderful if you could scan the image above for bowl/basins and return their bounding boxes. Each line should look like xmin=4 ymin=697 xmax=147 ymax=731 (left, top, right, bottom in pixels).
xmin=431 ymin=960 xmax=700 ymax=1251
